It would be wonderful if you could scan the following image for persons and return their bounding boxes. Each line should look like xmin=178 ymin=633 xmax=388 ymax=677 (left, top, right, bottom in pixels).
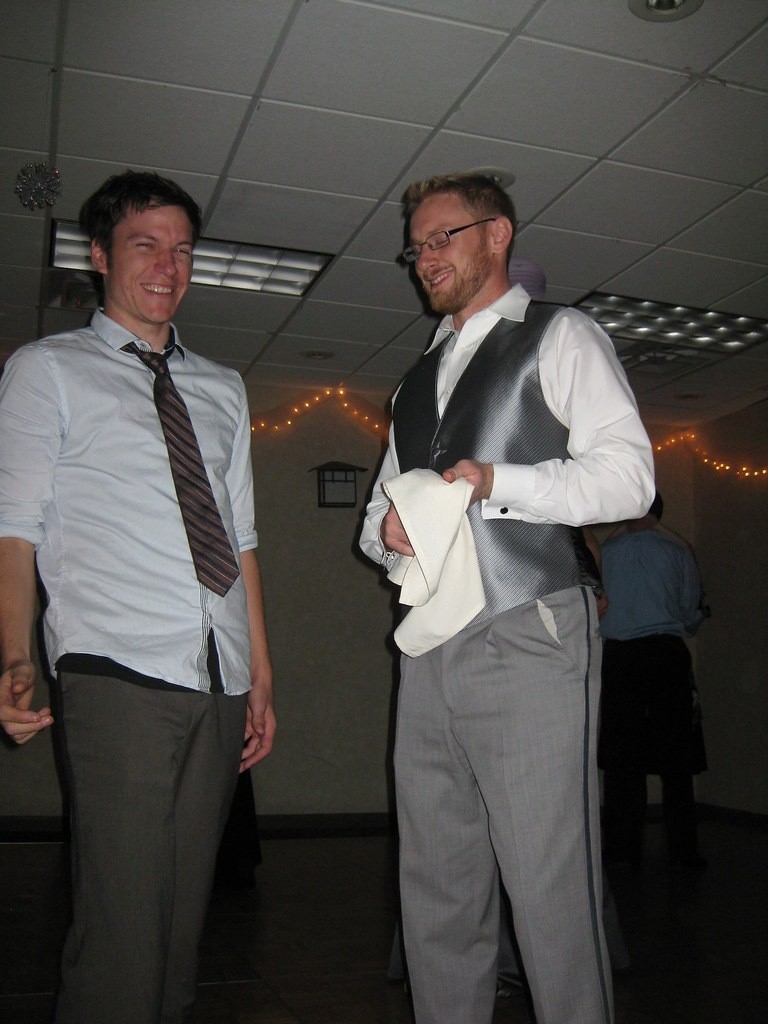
xmin=573 ymin=523 xmax=608 ymax=615
xmin=2 ymin=168 xmax=278 ymax=1022
xmin=346 ymin=175 xmax=659 ymax=1023
xmin=598 ymin=487 xmax=708 ymax=892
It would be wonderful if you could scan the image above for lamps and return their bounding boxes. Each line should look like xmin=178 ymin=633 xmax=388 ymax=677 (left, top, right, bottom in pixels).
xmin=15 ymin=160 xmax=62 ymax=213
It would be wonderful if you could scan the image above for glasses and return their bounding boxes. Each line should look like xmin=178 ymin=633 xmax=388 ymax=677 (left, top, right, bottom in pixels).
xmin=401 ymin=218 xmax=495 ymax=262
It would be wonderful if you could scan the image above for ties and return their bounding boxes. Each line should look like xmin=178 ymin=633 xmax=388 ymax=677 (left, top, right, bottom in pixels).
xmin=120 ymin=340 xmax=240 ymax=597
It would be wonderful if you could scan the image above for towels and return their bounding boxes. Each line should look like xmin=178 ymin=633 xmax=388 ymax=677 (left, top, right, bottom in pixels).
xmin=380 ymin=468 xmax=488 ymax=661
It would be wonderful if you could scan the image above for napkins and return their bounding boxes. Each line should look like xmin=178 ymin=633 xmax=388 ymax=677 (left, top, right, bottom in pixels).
xmin=379 ymin=467 xmax=486 ymax=660
